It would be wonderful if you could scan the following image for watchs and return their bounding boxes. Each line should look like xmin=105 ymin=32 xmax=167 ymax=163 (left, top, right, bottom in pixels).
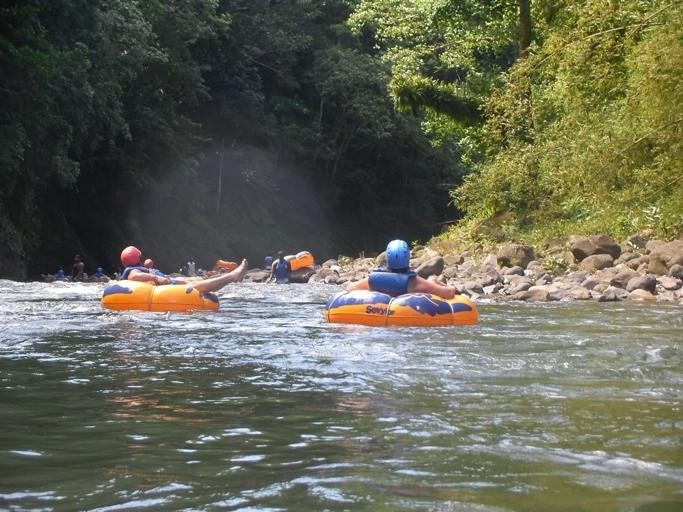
xmin=344 ymin=240 xmax=455 ymax=302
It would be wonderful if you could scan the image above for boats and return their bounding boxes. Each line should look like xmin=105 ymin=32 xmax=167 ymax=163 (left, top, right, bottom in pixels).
xmin=284 ymin=251 xmax=314 ymax=271
xmin=216 ymin=260 xmax=237 ymax=271
xmin=101 ymin=280 xmax=219 ymax=312
xmin=324 ymin=290 xmax=478 ymax=327
xmin=44 ymin=277 xmax=111 ymax=285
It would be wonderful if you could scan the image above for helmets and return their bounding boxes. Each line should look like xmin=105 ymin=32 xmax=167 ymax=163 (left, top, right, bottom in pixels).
xmin=120 ymin=246 xmax=142 ymax=266
xmin=387 ymin=239 xmax=410 ymax=270
xmin=144 ymin=259 xmax=153 ymax=269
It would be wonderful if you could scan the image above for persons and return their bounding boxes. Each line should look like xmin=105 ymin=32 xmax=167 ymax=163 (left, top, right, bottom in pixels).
xmin=69 ymin=254 xmax=84 ymax=282
xmin=52 ymin=269 xmax=68 ymax=283
xmin=90 ymin=266 xmax=108 ymax=279
xmin=111 ymin=267 xmax=121 ymax=281
xmin=185 ymin=255 xmax=196 ymax=276
xmin=143 ymin=258 xmax=172 ymax=277
xmin=120 ymin=246 xmax=248 ymax=292
xmin=266 ymin=250 xmax=289 ymax=285
